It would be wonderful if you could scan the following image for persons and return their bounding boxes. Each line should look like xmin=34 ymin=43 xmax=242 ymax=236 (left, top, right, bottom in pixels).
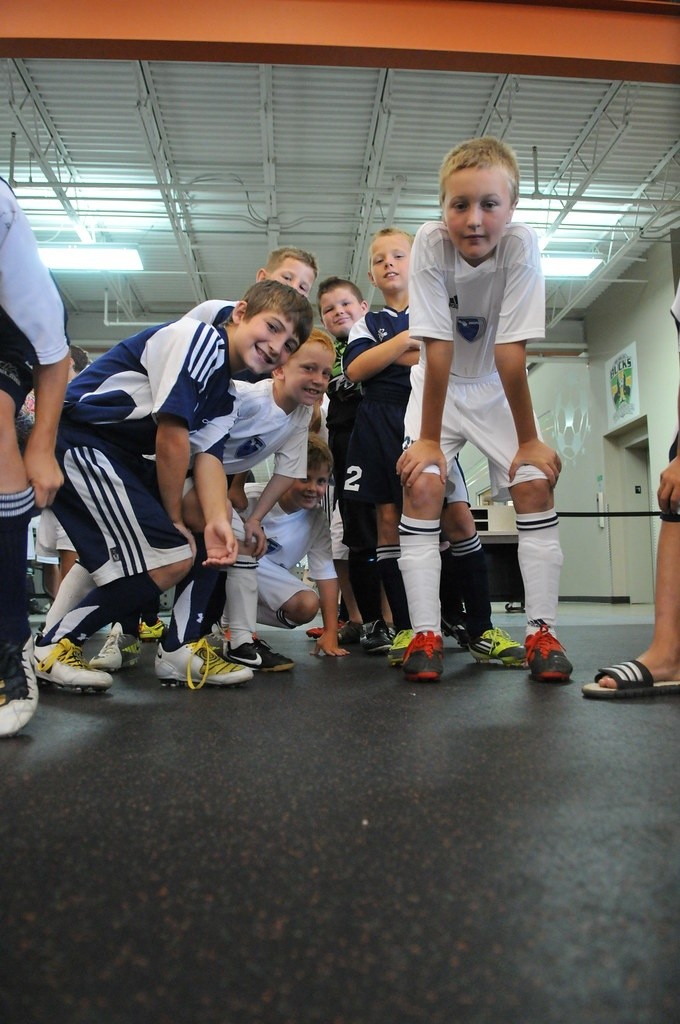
xmin=21 ymin=246 xmax=395 ymax=671
xmin=32 ymin=279 xmax=312 ymax=694
xmin=341 ymin=227 xmax=528 ymax=666
xmin=579 ymin=282 xmax=680 ymax=694
xmin=392 ymin=135 xmax=574 ymax=682
xmin=317 ymin=277 xmax=472 ymax=653
xmin=0 ymin=177 xmax=71 ymax=739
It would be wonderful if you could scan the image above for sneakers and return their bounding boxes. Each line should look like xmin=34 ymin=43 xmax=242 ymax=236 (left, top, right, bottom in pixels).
xmin=468 ymin=629 xmax=526 ymax=666
xmin=522 ymin=625 xmax=572 ymax=680
xmin=89 ymin=632 xmax=142 ymax=670
xmin=32 ymin=633 xmax=113 ymax=694
xmin=404 ymin=632 xmax=444 ymax=681
xmin=156 ymin=639 xmax=254 ymax=690
xmin=388 ymin=629 xmax=412 ymax=665
xmin=0 ymin=632 xmax=36 ymax=734
xmin=140 ymin=619 xmax=170 ymax=639
xmin=216 ymin=637 xmax=294 ymax=671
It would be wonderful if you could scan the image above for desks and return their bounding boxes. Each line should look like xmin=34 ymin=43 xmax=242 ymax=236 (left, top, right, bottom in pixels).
xmin=480 ymin=530 xmax=525 ymax=613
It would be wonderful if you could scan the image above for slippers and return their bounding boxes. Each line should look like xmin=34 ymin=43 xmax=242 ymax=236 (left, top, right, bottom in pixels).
xmin=581 ymin=658 xmax=680 ymax=697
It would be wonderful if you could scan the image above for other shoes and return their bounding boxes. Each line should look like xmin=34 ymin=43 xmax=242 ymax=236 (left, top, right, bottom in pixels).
xmin=360 ymin=620 xmax=393 ymax=656
xmin=307 ymin=616 xmax=359 ymax=645
xmin=441 ymin=613 xmax=469 ymax=649
